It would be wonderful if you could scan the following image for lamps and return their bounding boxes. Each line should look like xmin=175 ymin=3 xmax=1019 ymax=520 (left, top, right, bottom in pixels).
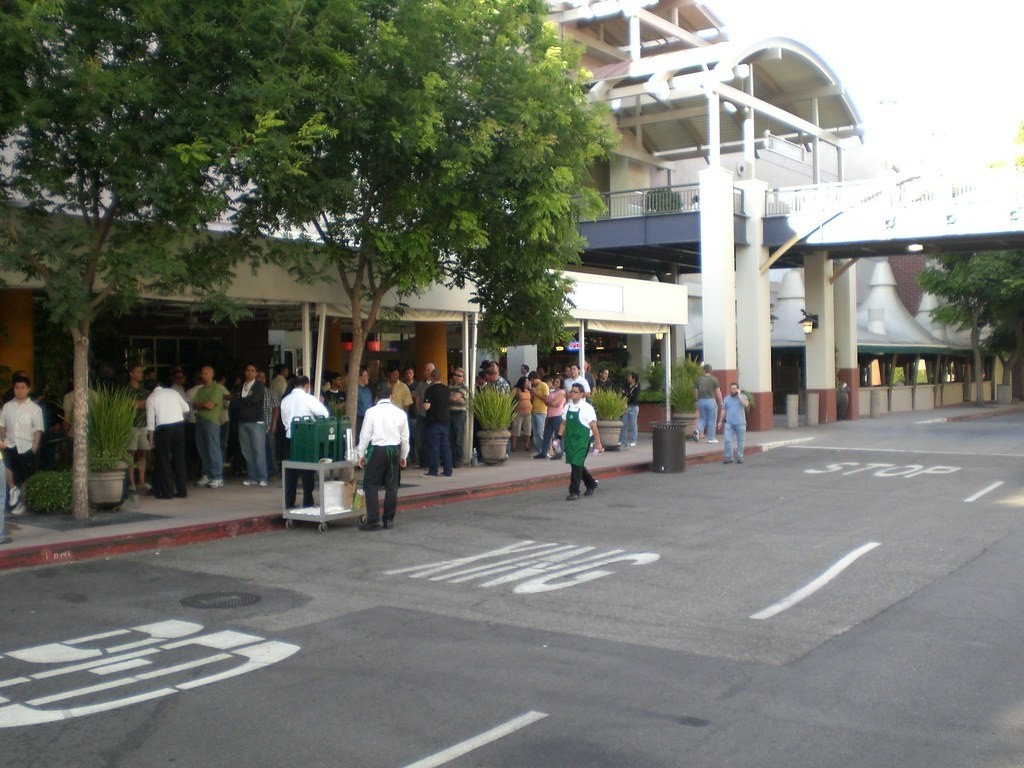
xmin=341 ymin=333 xmax=352 ymax=352
xmin=798 ymin=308 xmax=818 ymax=334
xmin=770 ymin=304 xmax=778 ymax=331
xmin=367 ymin=332 xmax=378 ymax=352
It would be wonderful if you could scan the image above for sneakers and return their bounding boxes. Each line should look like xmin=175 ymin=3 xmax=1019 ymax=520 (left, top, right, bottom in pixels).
xmin=359 ymin=521 xmax=382 ymax=531
xmin=384 ymin=520 xmax=393 ymax=528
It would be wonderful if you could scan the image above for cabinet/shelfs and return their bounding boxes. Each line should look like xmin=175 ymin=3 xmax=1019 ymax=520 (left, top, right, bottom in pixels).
xmin=282 ymin=460 xmax=368 ymax=534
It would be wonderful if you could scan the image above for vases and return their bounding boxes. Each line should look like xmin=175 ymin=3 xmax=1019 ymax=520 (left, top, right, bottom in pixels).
xmin=837 ymin=390 xmax=849 ymax=421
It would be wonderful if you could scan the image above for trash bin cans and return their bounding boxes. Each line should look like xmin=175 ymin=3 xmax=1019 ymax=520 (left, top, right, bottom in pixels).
xmin=651 ymin=420 xmax=688 ymax=472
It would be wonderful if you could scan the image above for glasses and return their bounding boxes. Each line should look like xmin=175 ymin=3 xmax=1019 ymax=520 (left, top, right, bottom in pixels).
xmin=453 ymin=374 xmax=462 ymax=378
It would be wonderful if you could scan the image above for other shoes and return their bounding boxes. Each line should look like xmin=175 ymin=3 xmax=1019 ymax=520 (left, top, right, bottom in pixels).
xmin=128 ymin=484 xmax=136 ymax=491
xmin=693 ymin=430 xmax=699 ymax=442
xmin=138 ymin=483 xmax=152 ymax=490
xmin=550 ymin=455 xmax=562 ymax=460
xmin=421 ymin=472 xmax=438 ymax=478
xmin=259 ymin=481 xmax=267 ymax=486
xmin=629 ymin=441 xmax=637 ymax=447
xmin=243 ymin=479 xmax=258 ymax=485
xmin=708 ymin=439 xmax=719 ymax=444
xmin=8 ymin=486 xmax=21 ymax=507
xmin=618 ymin=442 xmax=623 ymax=446
xmin=11 ymin=503 xmax=25 ymax=514
xmin=533 ymin=454 xmax=545 ymax=459
xmin=440 ymin=471 xmax=450 ymax=477
xmin=208 ymin=480 xmax=224 ymax=488
xmin=195 ymin=475 xmax=212 ymax=486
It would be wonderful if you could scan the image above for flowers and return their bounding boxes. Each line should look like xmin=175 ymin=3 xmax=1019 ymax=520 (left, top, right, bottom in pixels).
xmin=836 ymin=376 xmax=851 ymax=393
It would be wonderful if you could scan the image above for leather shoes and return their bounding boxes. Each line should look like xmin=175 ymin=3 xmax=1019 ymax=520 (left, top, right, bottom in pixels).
xmin=567 ymin=492 xmax=579 ymax=501
xmin=584 ymin=480 xmax=599 ymax=496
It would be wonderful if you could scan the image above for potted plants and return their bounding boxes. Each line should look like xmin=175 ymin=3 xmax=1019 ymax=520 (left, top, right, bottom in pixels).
xmin=669 ymin=353 xmax=704 ymax=437
xmin=636 ymin=390 xmax=666 ymax=432
xmin=590 ymin=385 xmax=632 ymax=448
xmin=464 ymin=383 xmax=529 ymax=459
xmin=46 ymin=377 xmax=147 ymax=511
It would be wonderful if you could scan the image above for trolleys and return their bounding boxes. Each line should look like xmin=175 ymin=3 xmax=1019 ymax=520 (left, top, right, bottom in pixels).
xmin=281 ymin=459 xmax=368 ymax=532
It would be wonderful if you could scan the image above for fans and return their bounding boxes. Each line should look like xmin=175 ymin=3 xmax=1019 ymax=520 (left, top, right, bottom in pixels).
xmin=156 ymin=307 xmax=231 ymax=329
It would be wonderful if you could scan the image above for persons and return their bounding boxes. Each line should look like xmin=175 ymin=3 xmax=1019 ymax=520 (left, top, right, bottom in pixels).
xmin=358 ymin=381 xmax=410 ymax=531
xmin=470 ymin=359 xmax=615 ymax=460
xmin=48 ymin=362 xmax=347 ymax=493
xmin=717 ymin=382 xmax=749 ymax=464
xmin=280 ymin=375 xmax=330 ymax=509
xmin=356 ymin=362 xmax=468 ymax=491
xmin=692 ymin=364 xmax=724 ymax=444
xmin=616 ymin=371 xmax=641 ymax=447
xmin=552 ymin=382 xmax=603 ymax=500
xmin=0 ymin=365 xmax=50 ymax=544
